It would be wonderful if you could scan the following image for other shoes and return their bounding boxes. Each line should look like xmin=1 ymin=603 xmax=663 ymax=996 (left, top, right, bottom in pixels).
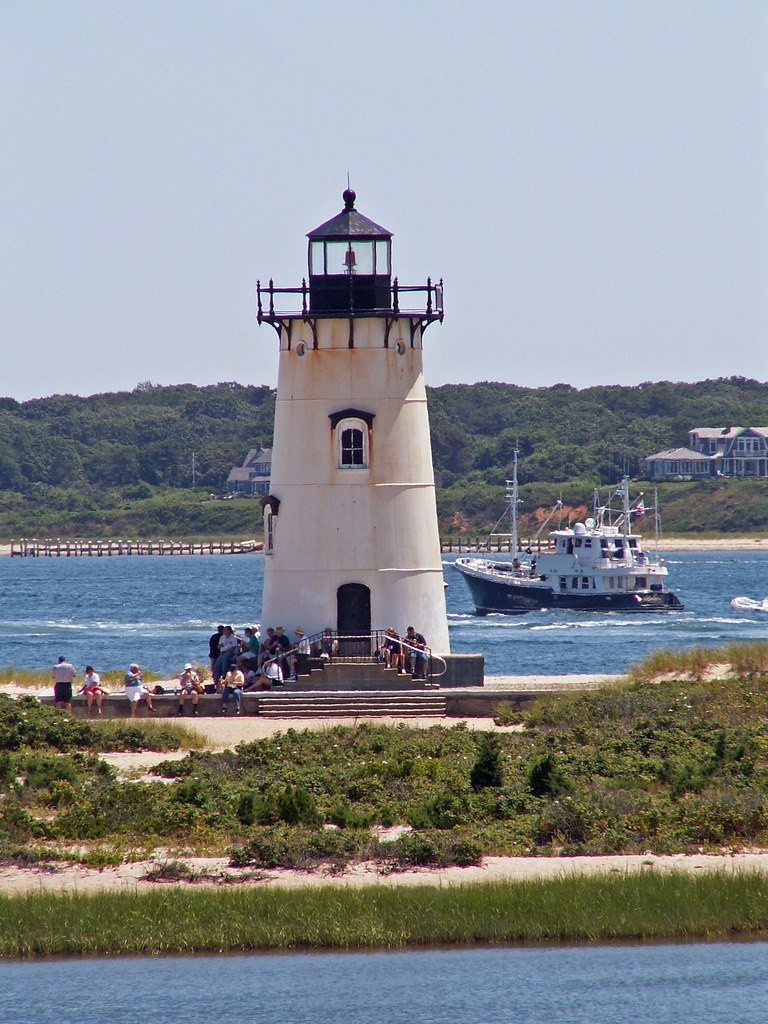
xmin=244 ymin=687 xmax=256 ymax=692
xmin=236 ymin=708 xmax=240 ymax=715
xmin=401 ymin=668 xmax=406 ymax=674
xmin=320 ymin=652 xmax=329 ymax=659
xmin=193 ymin=708 xmax=199 ymax=714
xmin=287 ymin=675 xmax=296 ymax=680
xmin=132 ymin=713 xmax=135 ymax=718
xmin=411 ymin=669 xmax=415 ymax=676
xmin=87 ymin=709 xmax=91 ymax=714
xmin=221 ymin=707 xmax=227 ymax=713
xmin=422 ymin=673 xmax=427 ymax=678
xmin=99 ymin=708 xmax=101 ymax=714
xmin=148 ymin=707 xmax=157 ymax=712
xmin=178 ymin=709 xmax=183 ymax=716
xmin=387 ymin=664 xmax=390 ymax=668
xmin=63 ymin=718 xmax=69 ymax=722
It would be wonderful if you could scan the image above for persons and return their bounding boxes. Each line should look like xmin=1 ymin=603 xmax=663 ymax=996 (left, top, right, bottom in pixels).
xmin=51 ymin=656 xmax=76 ymax=713
xmin=315 ymin=628 xmax=338 ymax=658
xmin=244 ymin=656 xmax=283 ymax=692
xmin=403 ymin=626 xmax=428 ymax=679
xmin=77 ymin=666 xmax=103 ymax=714
xmin=219 ymin=664 xmax=244 ymax=715
xmin=378 ymin=627 xmax=406 ymax=674
xmin=285 ymin=625 xmax=311 ymax=679
xmin=124 ymin=663 xmax=157 ymax=718
xmin=208 ymin=625 xmax=289 ymax=685
xmin=177 ymin=663 xmax=200 ymax=716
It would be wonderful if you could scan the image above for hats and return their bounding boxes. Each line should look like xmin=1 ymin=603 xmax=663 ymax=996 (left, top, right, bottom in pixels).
xmin=185 ymin=663 xmax=193 ymax=670
xmin=295 ymin=627 xmax=304 ymax=635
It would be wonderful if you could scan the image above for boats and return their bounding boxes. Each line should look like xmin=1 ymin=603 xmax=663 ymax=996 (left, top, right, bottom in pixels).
xmin=730 ymin=596 xmax=768 ymax=614
xmin=453 ymin=435 xmax=686 ymax=615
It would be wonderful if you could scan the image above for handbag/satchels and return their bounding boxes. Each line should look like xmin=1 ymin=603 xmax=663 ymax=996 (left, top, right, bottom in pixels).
xmin=205 ymin=684 xmax=217 ymax=694
xmin=153 ymin=686 xmax=164 ymax=694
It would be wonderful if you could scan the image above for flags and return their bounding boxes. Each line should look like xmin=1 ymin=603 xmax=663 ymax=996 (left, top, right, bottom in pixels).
xmin=636 ymin=501 xmax=645 ymax=516
